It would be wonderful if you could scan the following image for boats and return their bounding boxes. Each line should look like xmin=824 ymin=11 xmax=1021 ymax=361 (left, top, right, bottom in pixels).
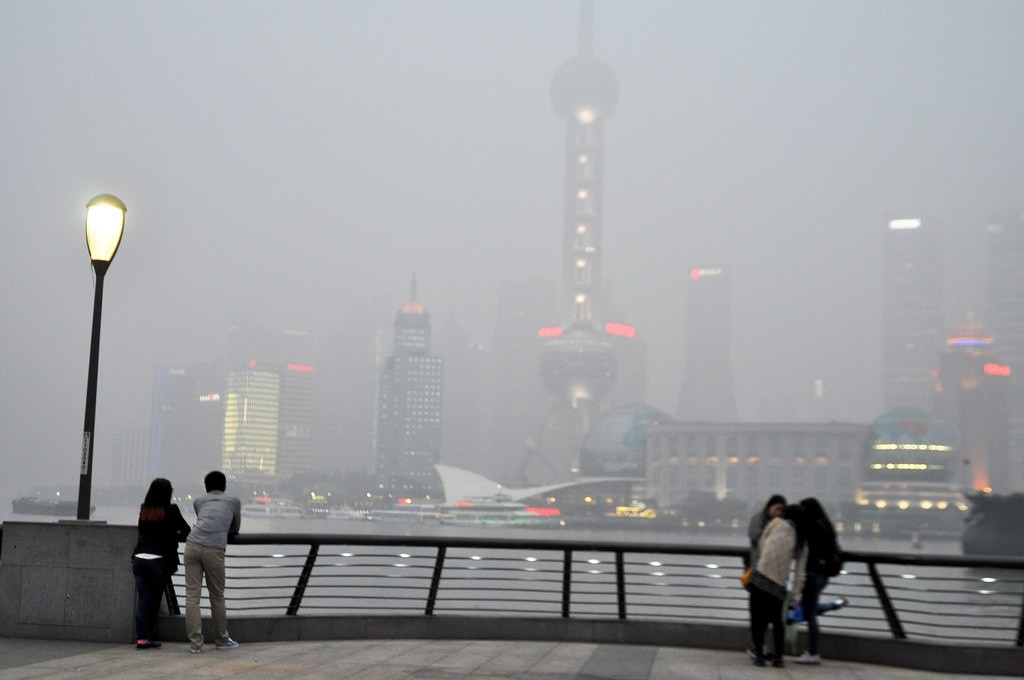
xmin=240 ymin=504 xmax=303 ymax=518
xmin=441 ymin=502 xmax=541 ymax=527
xmin=364 ymin=503 xmax=441 ymax=526
xmin=328 ymin=507 xmax=358 ymax=520
xmin=12 ymin=497 xmax=96 ymax=517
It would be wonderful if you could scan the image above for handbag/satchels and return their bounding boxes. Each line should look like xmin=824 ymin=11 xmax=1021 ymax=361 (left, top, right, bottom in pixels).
xmin=742 ymin=565 xmax=757 ymax=593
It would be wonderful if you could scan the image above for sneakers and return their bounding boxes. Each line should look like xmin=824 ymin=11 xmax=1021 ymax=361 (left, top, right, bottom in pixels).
xmin=795 ymin=651 xmax=822 ymax=665
xmin=189 ymin=647 xmax=200 ymax=652
xmin=217 ymin=637 xmax=238 ymax=649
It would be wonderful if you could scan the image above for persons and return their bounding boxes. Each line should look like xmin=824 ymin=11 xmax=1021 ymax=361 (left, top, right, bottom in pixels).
xmin=748 ymin=495 xmax=847 ymax=667
xmin=182 ymin=471 xmax=241 ymax=650
xmin=130 ymin=479 xmax=191 ymax=649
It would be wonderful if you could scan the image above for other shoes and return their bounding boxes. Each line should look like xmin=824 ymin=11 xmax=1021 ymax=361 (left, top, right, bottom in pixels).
xmin=136 ymin=640 xmax=162 ymax=649
xmin=754 ymin=647 xmax=782 ymax=667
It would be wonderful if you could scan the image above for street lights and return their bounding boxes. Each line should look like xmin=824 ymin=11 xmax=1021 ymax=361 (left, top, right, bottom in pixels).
xmin=77 ymin=194 xmax=127 ymax=521
